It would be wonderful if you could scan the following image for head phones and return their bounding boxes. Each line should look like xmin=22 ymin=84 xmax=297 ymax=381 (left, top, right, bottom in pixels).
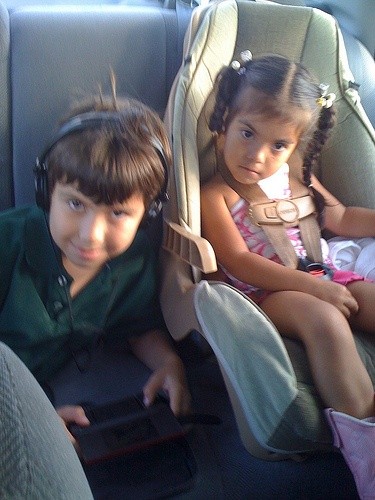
xmin=35 ymin=110 xmax=170 ymax=230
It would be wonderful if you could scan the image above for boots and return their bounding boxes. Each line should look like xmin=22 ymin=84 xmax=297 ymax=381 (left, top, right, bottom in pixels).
xmin=325 ymin=408 xmax=375 ymax=500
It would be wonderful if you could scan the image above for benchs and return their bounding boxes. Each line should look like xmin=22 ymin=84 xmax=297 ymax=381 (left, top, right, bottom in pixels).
xmin=0 ymin=2 xmax=375 ymax=437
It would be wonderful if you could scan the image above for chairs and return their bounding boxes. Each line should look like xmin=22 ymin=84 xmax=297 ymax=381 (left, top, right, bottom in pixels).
xmin=159 ymin=0 xmax=375 ymax=459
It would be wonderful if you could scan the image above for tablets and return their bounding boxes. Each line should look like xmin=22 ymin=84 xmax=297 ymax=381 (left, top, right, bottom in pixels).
xmin=72 ymin=403 xmax=191 ymax=466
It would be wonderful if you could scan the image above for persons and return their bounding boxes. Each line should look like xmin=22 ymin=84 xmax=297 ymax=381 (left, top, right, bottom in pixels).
xmin=201 ymin=54 xmax=375 ymax=500
xmin=0 ymin=94 xmax=190 ymax=455
